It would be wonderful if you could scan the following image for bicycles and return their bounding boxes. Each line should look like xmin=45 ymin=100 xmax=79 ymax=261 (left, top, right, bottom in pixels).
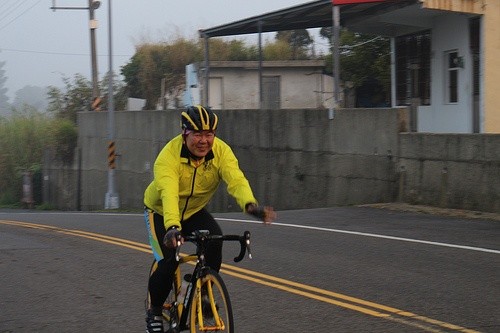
xmin=146 ymin=231 xmax=254 ymax=333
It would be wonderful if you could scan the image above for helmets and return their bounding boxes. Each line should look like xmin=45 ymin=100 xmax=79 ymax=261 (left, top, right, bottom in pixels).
xmin=179 ymin=104 xmax=218 ymax=132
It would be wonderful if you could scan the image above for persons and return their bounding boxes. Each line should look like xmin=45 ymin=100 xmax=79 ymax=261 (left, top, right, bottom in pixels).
xmin=144 ymin=105 xmax=277 ymax=333
xmin=156 ymin=83 xmax=186 ymax=109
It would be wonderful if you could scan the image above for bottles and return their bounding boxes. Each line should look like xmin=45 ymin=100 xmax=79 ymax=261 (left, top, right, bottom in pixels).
xmin=176 ymin=273 xmax=193 ymax=304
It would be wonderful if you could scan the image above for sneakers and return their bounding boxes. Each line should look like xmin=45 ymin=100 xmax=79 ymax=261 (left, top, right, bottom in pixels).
xmin=146 ymin=310 xmax=164 ymax=333
xmin=202 ymin=300 xmax=219 ymax=318
